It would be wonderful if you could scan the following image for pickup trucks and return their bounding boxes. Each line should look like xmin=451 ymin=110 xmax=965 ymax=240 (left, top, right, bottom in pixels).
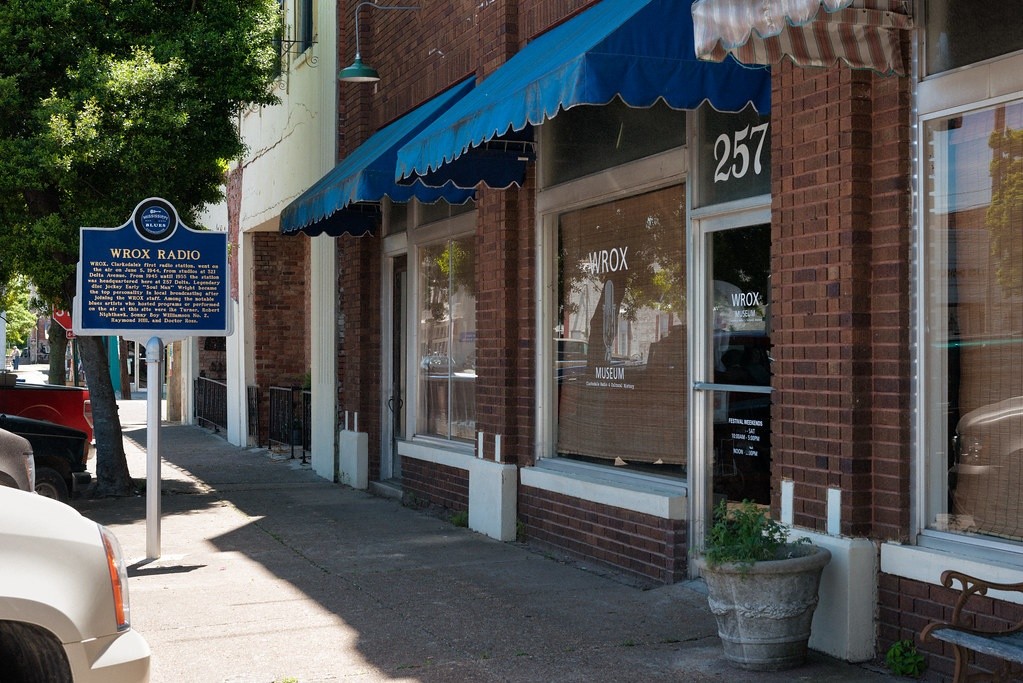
xmin=0 ymin=379 xmax=92 ymax=499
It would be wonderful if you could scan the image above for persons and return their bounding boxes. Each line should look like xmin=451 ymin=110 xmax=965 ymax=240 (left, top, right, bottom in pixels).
xmin=11 ymin=346 xmax=20 ymax=370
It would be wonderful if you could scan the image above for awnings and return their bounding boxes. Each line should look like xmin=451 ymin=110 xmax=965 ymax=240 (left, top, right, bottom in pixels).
xmin=690 ymin=0 xmax=912 ymax=80
xmin=394 ymin=0 xmax=772 ymax=195
xmin=278 ymin=74 xmax=477 ymax=239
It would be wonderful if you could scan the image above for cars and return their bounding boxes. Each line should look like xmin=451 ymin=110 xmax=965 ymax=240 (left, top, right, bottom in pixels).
xmin=1 ymin=430 xmax=37 ymax=493
xmin=948 ymin=396 xmax=1023 ymax=535
xmin=421 ymin=355 xmax=463 ymax=372
xmin=0 ymin=483 xmax=133 ymax=683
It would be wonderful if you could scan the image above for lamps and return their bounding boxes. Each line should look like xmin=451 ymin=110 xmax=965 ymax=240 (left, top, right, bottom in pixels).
xmin=337 ymin=2 xmax=421 ymax=81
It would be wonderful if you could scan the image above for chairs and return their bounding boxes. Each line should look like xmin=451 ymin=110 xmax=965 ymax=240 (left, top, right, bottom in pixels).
xmin=920 ymin=570 xmax=1023 ymax=683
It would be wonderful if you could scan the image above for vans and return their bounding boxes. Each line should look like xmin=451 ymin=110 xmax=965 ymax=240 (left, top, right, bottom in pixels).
xmin=557 ymin=338 xmax=630 ymax=368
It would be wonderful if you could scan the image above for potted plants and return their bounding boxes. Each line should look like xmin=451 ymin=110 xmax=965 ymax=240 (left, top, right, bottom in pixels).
xmin=689 ymin=498 xmax=832 ymax=671
xmin=281 ymin=416 xmax=302 ymax=444
